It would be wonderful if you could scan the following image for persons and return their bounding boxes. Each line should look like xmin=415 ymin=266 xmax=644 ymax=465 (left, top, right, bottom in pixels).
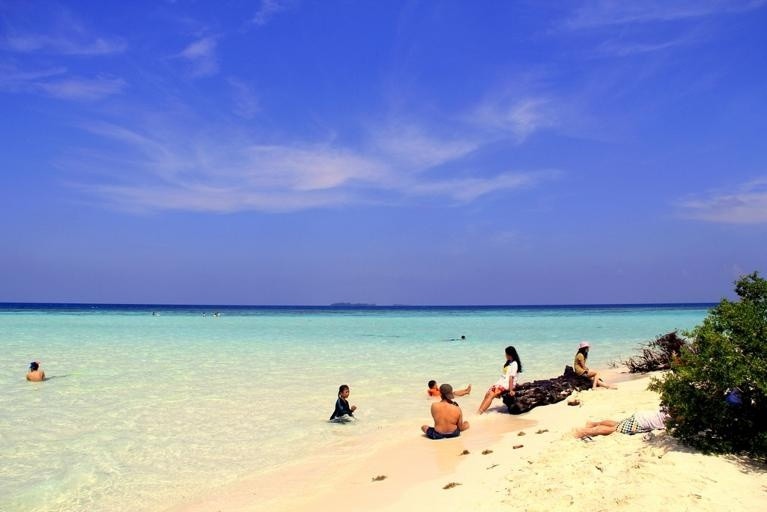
xmin=329 ymin=384 xmax=357 ymax=425
xmin=573 ymin=340 xmax=619 ymax=391
xmin=476 ymin=345 xmax=524 ymax=417
xmin=25 ymin=360 xmax=47 ymax=382
xmin=575 ymin=404 xmax=673 ymax=440
xmin=427 ymin=379 xmax=472 ymax=398
xmin=420 ymin=384 xmax=470 ymax=440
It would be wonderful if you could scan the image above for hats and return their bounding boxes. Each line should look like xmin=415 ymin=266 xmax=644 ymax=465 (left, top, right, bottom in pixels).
xmin=578 ymin=341 xmax=591 ymax=350
